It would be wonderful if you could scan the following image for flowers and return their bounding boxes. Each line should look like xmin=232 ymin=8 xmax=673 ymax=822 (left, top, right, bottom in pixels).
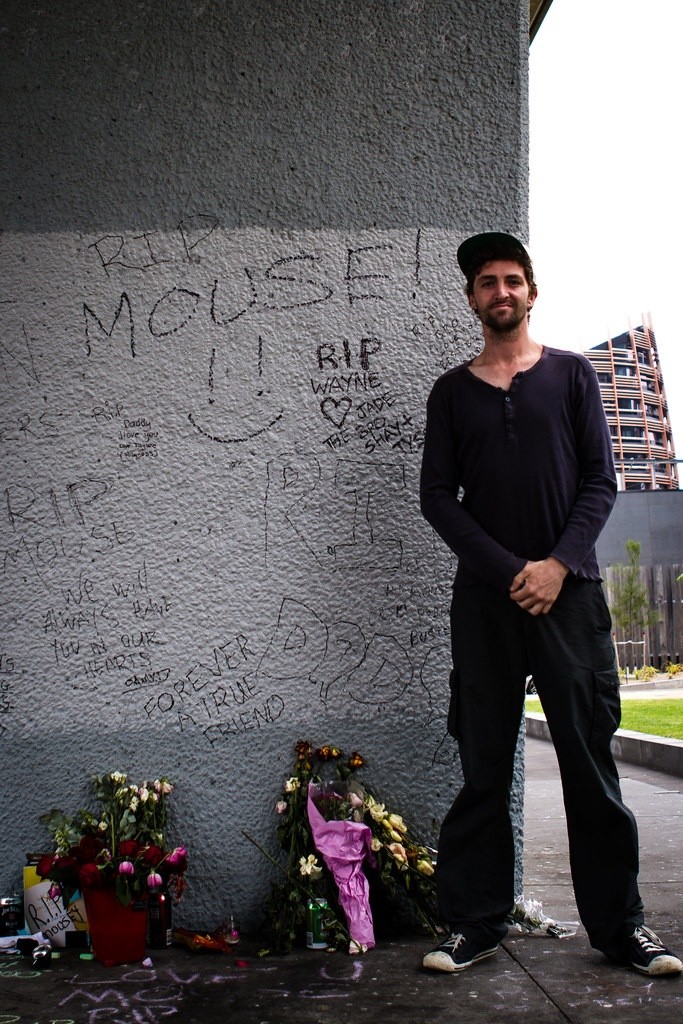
xmin=37 ymin=770 xmax=190 ymax=910
xmin=241 ymin=738 xmax=573 ymax=956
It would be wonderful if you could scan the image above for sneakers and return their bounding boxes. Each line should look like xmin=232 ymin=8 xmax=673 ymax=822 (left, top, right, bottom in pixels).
xmin=421 ymin=931 xmax=500 ymax=972
xmin=601 ymin=925 xmax=683 ymax=977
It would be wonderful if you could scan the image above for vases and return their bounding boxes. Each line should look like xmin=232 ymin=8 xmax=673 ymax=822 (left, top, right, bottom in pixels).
xmin=80 ymin=882 xmax=148 ymax=964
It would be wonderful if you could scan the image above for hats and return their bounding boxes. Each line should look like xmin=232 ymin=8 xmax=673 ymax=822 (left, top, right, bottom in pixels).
xmin=457 ymin=232 xmax=528 ymax=277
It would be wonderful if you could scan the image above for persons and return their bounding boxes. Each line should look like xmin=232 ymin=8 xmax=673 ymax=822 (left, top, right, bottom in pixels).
xmin=418 ymin=232 xmax=683 ymax=977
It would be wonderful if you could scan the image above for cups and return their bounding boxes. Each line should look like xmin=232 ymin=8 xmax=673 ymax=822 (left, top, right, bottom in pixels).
xmin=223 ymin=920 xmax=240 ymax=943
